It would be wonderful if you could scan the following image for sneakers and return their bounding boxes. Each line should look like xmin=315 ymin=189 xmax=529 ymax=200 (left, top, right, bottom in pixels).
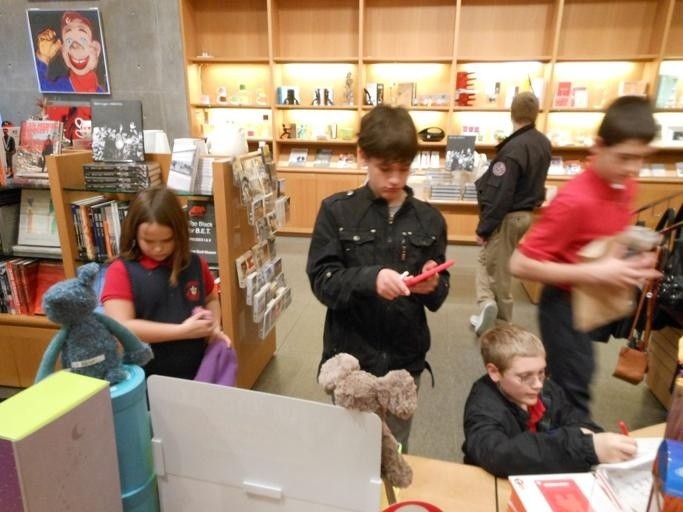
xmin=470 ymin=302 xmax=498 ymax=337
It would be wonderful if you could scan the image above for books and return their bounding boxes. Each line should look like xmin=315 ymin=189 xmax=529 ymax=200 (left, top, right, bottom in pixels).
xmin=429 ymin=136 xmax=480 ymax=200
xmin=554 ymin=80 xmax=649 ymax=109
xmin=181 ymin=200 xmax=219 ymax=269
xmin=232 ymin=151 xmax=290 ymax=339
xmin=1 ymin=259 xmax=65 ymax=316
xmin=83 ymin=99 xmax=161 ymax=191
xmin=507 ymin=429 xmax=665 ymax=512
xmin=1 ymin=188 xmax=63 ymax=259
xmin=1 ymin=121 xmax=63 ymax=178
xmin=70 ymin=195 xmax=134 ymax=263
xmin=312 ymin=88 xmax=334 ymax=104
xmin=366 ymin=82 xmax=417 ymax=105
xmin=167 ymin=138 xmax=208 ymax=193
xmin=311 ymin=123 xmax=355 ymax=169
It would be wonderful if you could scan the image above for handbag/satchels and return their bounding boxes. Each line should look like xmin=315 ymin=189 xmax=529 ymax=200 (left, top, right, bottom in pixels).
xmin=589 ymin=205 xmax=683 ymax=344
xmin=615 ymin=342 xmax=650 ymax=385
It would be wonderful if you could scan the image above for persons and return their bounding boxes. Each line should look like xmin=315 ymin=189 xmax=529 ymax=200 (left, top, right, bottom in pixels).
xmin=29 ymin=11 xmax=108 ymax=93
xmin=100 ymin=190 xmax=237 ymax=386
xmin=461 ymin=323 xmax=638 ymax=480
xmin=508 ymin=95 xmax=663 ymax=435
xmin=305 ymin=104 xmax=453 ymax=455
xmin=470 ymin=90 xmax=552 ymax=339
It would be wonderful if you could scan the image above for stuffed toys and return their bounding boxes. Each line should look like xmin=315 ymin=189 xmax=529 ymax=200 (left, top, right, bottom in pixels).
xmin=34 ymin=263 xmax=155 ymax=389
xmin=317 ymin=352 xmax=418 ymax=488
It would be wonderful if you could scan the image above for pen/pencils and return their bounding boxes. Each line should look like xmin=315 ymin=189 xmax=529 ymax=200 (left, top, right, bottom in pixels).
xmin=399 ymin=271 xmax=410 ymax=281
xmin=618 ymin=420 xmax=629 ymax=436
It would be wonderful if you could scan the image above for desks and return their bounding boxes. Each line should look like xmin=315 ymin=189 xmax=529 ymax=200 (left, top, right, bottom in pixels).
xmin=379 ymin=454 xmax=497 ymax=512
xmin=496 ymin=423 xmax=667 ymax=512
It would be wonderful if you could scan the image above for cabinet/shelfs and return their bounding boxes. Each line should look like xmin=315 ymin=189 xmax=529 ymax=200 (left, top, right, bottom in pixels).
xmin=2 ymin=122 xmax=280 ymax=389
xmin=177 ymin=2 xmax=682 ymax=251
